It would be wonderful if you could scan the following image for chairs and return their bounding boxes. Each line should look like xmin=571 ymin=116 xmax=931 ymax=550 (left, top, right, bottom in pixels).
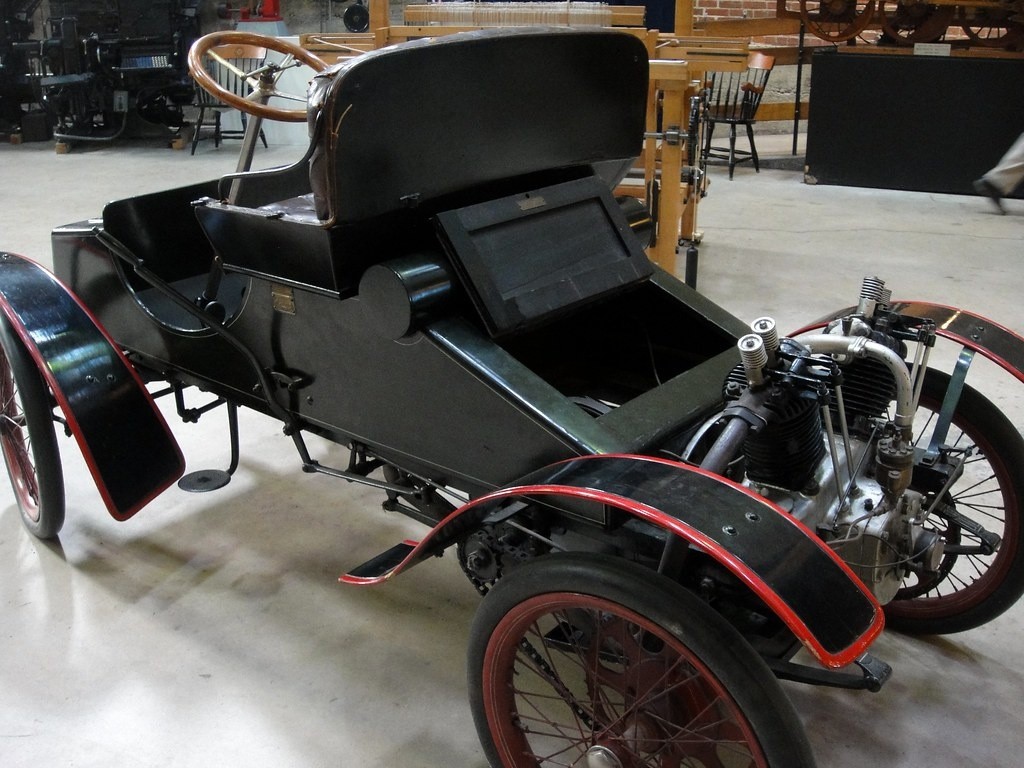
xmin=700 ymin=53 xmax=775 ymax=180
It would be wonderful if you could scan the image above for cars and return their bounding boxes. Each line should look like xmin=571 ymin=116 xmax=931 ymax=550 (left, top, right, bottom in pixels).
xmin=2 ymin=28 xmax=1024 ymax=768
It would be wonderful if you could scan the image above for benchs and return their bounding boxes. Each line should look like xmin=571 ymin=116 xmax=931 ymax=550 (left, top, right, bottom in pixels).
xmin=191 ymin=24 xmax=649 ymax=292
